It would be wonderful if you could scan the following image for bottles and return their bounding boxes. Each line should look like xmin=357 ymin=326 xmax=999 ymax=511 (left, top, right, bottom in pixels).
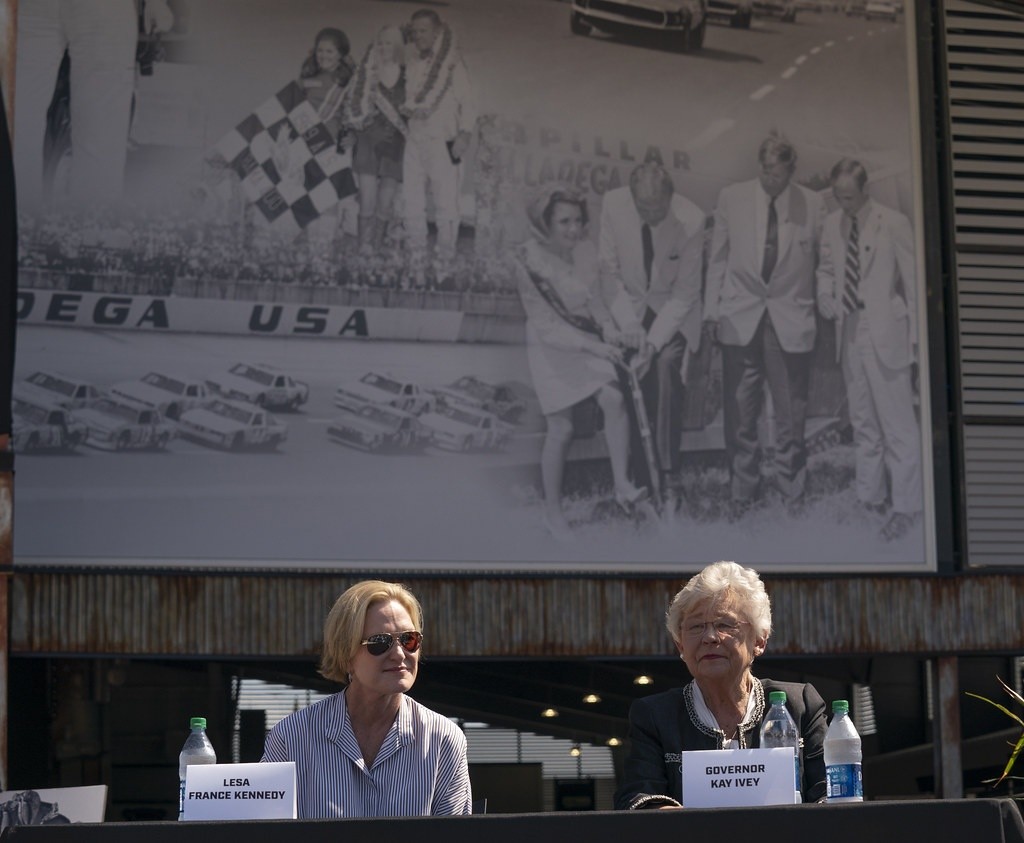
xmin=760 ymin=690 xmax=803 ymax=805
xmin=178 ymin=717 xmax=217 ymax=823
xmin=822 ymin=699 xmax=865 ymax=804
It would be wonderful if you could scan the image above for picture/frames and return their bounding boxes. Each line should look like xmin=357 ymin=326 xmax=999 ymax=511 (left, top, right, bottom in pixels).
xmin=0 ymin=0 xmax=954 ymax=578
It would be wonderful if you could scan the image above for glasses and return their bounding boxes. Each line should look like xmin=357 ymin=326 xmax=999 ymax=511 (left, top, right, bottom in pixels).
xmin=360 ymin=631 xmax=423 ymax=656
xmin=679 ymin=619 xmax=750 ymax=640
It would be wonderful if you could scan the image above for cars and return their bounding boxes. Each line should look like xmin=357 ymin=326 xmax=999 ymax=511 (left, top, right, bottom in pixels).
xmin=571 ymin=0 xmax=897 ymax=54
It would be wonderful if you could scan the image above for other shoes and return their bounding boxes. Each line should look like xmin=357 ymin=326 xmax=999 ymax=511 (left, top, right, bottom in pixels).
xmin=880 ymin=512 xmax=916 ymax=540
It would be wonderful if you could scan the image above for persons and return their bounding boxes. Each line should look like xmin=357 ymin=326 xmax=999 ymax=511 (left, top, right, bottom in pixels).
xmin=613 ymin=561 xmax=830 ymax=810
xmin=287 ymin=27 xmax=355 ymax=257
xmin=814 ymin=158 xmax=920 ymax=545
xmin=12 ymin=0 xmax=176 ymax=227
xmin=401 ymin=9 xmax=473 ymax=291
xmin=597 ymin=165 xmax=706 ymax=520
xmin=344 ymin=24 xmax=406 ymax=274
xmin=258 ymin=580 xmax=472 ymax=820
xmin=506 ymin=182 xmax=648 ymax=550
xmin=703 ymin=135 xmax=828 ymax=524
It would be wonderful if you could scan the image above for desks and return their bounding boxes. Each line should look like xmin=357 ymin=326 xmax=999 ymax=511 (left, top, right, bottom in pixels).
xmin=0 ymin=786 xmax=1024 ymax=843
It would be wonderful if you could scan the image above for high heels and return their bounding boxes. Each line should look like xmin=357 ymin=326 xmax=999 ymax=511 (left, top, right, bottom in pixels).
xmin=615 ymin=482 xmax=647 ymax=516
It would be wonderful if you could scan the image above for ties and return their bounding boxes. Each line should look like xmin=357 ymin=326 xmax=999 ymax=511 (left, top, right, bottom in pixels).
xmin=641 ymin=222 xmax=654 ymax=291
xmin=843 ymin=217 xmax=860 ymax=317
xmin=760 ymin=197 xmax=778 ymax=284
